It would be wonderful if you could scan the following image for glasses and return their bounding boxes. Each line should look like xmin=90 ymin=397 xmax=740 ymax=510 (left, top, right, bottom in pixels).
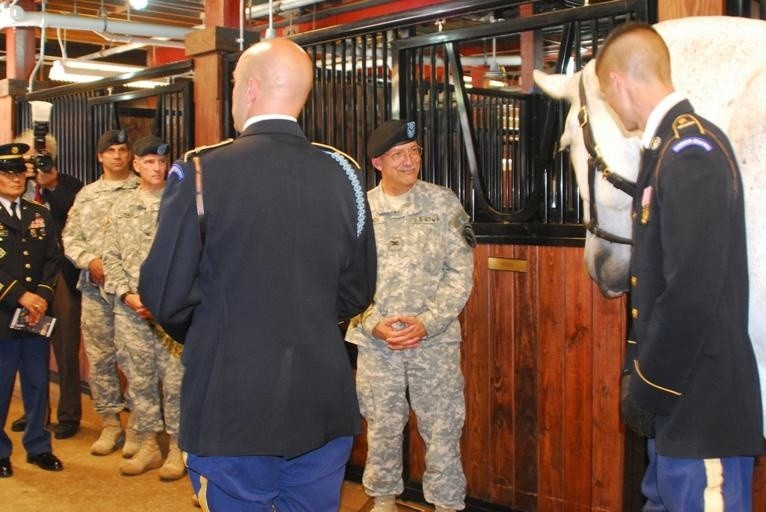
xmin=385 ymin=146 xmax=423 ymax=159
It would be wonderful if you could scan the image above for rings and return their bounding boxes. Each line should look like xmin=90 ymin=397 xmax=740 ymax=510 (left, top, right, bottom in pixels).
xmin=33 ymin=303 xmax=41 ymax=311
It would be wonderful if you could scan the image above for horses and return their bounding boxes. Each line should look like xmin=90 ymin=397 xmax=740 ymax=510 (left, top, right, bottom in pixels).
xmin=531 ymin=13 xmax=765 ymax=444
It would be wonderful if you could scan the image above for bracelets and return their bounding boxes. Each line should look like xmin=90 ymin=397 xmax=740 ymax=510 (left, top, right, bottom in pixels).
xmin=119 ymin=291 xmax=132 ymax=306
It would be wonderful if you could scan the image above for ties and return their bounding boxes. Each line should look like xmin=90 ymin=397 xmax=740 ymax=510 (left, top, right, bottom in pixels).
xmin=11 ymin=201 xmax=19 ymax=221
xmin=35 ymin=184 xmax=44 ymax=204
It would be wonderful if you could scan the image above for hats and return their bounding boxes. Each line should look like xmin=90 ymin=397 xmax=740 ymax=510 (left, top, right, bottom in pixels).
xmin=366 ymin=120 xmax=416 ymax=157
xmin=97 ymin=130 xmax=126 ymax=152
xmin=0 ymin=143 xmax=29 ymax=173
xmin=133 ymin=136 xmax=170 ymax=156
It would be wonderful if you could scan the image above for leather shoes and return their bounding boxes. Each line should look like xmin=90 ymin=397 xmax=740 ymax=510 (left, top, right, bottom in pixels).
xmin=0 ymin=412 xmax=80 ymax=476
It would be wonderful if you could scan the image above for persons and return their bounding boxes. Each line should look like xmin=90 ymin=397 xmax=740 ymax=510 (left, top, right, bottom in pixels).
xmin=61 ymin=127 xmax=143 ymax=459
xmin=0 ymin=141 xmax=65 ymax=477
xmin=13 ymin=126 xmax=87 ymax=438
xmin=346 ymin=117 xmax=477 ymax=511
xmin=594 ymin=20 xmax=766 ymax=512
xmin=137 ymin=39 xmax=378 ymax=510
xmin=104 ymin=132 xmax=188 ymax=481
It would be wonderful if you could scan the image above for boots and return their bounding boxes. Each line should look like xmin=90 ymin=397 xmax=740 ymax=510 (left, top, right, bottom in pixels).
xmin=370 ymin=496 xmax=398 ymax=512
xmin=91 ymin=411 xmax=185 ymax=480
